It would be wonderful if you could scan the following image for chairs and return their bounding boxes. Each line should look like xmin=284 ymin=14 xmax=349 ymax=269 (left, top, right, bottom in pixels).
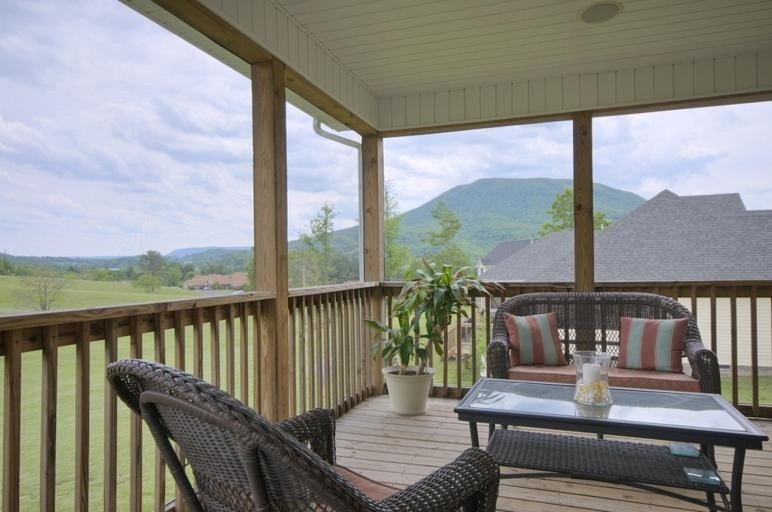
xmin=106 ymin=359 xmax=499 ymax=512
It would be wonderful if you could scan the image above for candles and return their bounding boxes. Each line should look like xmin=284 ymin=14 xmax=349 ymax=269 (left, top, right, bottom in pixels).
xmin=583 ymin=364 xmax=601 ymax=387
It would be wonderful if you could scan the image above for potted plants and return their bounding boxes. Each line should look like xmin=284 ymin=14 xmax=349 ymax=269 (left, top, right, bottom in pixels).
xmin=364 ymin=260 xmax=506 ymax=415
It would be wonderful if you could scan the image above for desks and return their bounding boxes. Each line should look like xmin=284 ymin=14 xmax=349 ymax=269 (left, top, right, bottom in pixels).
xmin=453 ymin=378 xmax=769 ymax=512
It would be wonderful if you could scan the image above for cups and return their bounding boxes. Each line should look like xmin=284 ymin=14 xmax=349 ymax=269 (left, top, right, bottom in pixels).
xmin=572 ymin=350 xmax=615 ymax=406
xmin=582 ymin=362 xmax=600 ymax=385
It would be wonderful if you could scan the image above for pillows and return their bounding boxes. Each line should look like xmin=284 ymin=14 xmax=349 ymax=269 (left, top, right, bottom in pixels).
xmin=615 ymin=317 xmax=690 ymax=374
xmin=502 ymin=311 xmax=567 ymax=367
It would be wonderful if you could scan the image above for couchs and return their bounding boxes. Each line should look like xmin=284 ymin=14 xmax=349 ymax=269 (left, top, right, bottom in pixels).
xmin=486 ymin=292 xmax=721 ymax=416
